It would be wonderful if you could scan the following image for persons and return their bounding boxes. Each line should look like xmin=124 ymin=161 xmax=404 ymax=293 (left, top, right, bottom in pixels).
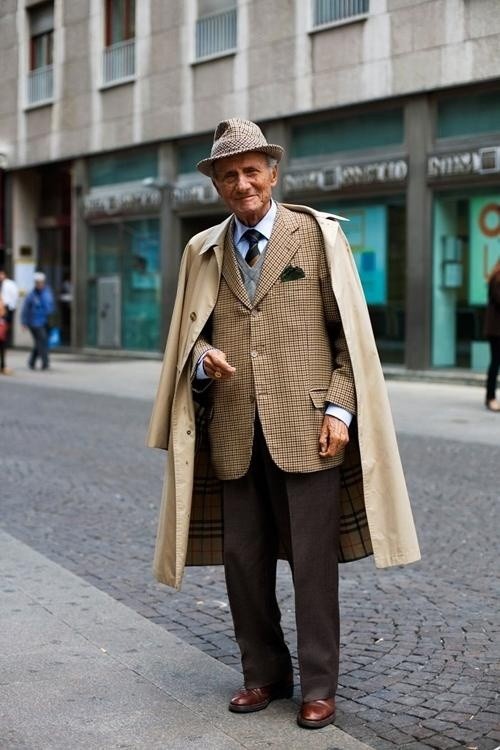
xmin=147 ymin=120 xmax=424 ymax=731
xmin=20 ymin=272 xmax=59 ymax=371
xmin=484 ymin=259 xmax=500 ymax=413
xmin=0 ymin=264 xmax=19 ymax=375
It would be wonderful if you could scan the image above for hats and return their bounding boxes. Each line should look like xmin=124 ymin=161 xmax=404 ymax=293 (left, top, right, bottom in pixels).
xmin=31 ymin=271 xmax=47 ymax=282
xmin=196 ymin=118 xmax=284 ymax=176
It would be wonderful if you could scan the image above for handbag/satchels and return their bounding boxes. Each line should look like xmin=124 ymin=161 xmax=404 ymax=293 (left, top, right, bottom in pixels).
xmin=47 ymin=313 xmax=63 ymax=329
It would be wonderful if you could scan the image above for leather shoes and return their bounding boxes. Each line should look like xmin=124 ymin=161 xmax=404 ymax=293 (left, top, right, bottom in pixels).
xmin=229 ymin=670 xmax=293 ymax=713
xmin=297 ymin=695 xmax=336 ymax=728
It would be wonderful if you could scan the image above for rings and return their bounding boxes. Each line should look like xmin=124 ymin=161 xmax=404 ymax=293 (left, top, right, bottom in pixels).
xmin=215 ymin=372 xmax=222 ymax=378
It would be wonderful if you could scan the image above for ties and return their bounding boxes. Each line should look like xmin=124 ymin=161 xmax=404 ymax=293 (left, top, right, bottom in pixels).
xmin=242 ymin=229 xmax=264 ymax=267
